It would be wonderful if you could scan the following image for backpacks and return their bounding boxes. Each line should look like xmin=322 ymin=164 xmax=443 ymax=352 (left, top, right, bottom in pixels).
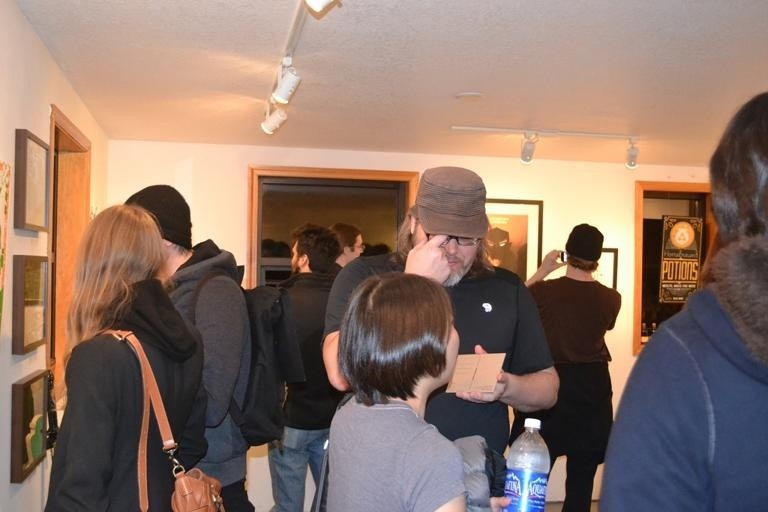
xmin=188 ymin=267 xmax=306 ymax=447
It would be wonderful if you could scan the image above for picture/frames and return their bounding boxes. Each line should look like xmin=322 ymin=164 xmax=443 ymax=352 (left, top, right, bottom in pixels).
xmin=11 ymin=370 xmax=47 ymax=485
xmin=591 ymin=248 xmax=618 ymax=291
xmin=13 ymin=254 xmax=48 ymax=355
xmin=14 ymin=128 xmax=52 ymax=234
xmin=474 ymin=199 xmax=543 ymax=286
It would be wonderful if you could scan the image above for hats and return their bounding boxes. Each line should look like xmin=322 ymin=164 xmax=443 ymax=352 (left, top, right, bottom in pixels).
xmin=416 ymin=166 xmax=489 ymax=238
xmin=566 ymin=223 xmax=605 ymax=261
xmin=124 ymin=185 xmax=193 ymax=249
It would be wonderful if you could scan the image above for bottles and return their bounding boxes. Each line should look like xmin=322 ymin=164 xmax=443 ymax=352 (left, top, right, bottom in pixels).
xmin=502 ymin=418 xmax=550 ymax=512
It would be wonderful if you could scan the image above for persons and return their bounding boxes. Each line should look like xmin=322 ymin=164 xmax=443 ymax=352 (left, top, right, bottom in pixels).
xmin=44 ymin=205 xmax=208 ymax=511
xmin=123 ymin=184 xmax=255 ymax=511
xmin=360 ymin=243 xmax=391 ymax=256
xmin=325 ymin=271 xmax=512 ymax=511
xmin=329 ymin=223 xmax=365 ymax=275
xmin=265 ymin=222 xmax=345 ymax=511
xmin=262 ymin=238 xmax=291 ymax=287
xmin=598 ymin=93 xmax=768 ymax=511
xmin=321 ymin=166 xmax=560 ymax=455
xmin=509 ymin=223 xmax=622 ymax=512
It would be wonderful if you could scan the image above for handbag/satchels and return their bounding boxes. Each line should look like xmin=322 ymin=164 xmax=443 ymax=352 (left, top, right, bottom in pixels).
xmin=94 ymin=325 xmax=225 ymax=512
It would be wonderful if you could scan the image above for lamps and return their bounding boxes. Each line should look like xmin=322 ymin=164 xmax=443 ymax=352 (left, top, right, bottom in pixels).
xmin=272 ymin=58 xmax=300 ymax=105
xmin=625 ymin=139 xmax=640 ymax=171
xmin=305 ymin=1 xmax=336 ymax=14
xmin=520 ymin=132 xmax=539 ymax=165
xmin=260 ymin=103 xmax=286 ymax=136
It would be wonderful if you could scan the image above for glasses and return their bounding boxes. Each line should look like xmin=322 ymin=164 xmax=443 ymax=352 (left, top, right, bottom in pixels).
xmin=351 ymin=243 xmax=366 ymax=251
xmin=418 ymin=220 xmax=479 ymax=248
